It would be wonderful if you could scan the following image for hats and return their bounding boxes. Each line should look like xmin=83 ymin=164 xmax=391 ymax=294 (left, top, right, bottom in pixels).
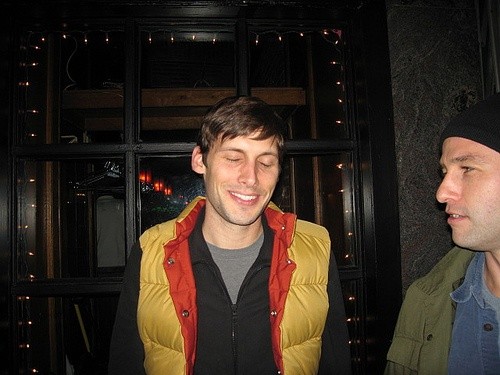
xmin=437 ymin=91 xmax=499 ymax=154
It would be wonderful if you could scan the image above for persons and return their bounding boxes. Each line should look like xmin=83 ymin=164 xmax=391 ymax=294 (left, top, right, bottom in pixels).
xmin=114 ymin=96 xmax=350 ymax=375
xmin=384 ymin=111 xmax=499 ymax=375
